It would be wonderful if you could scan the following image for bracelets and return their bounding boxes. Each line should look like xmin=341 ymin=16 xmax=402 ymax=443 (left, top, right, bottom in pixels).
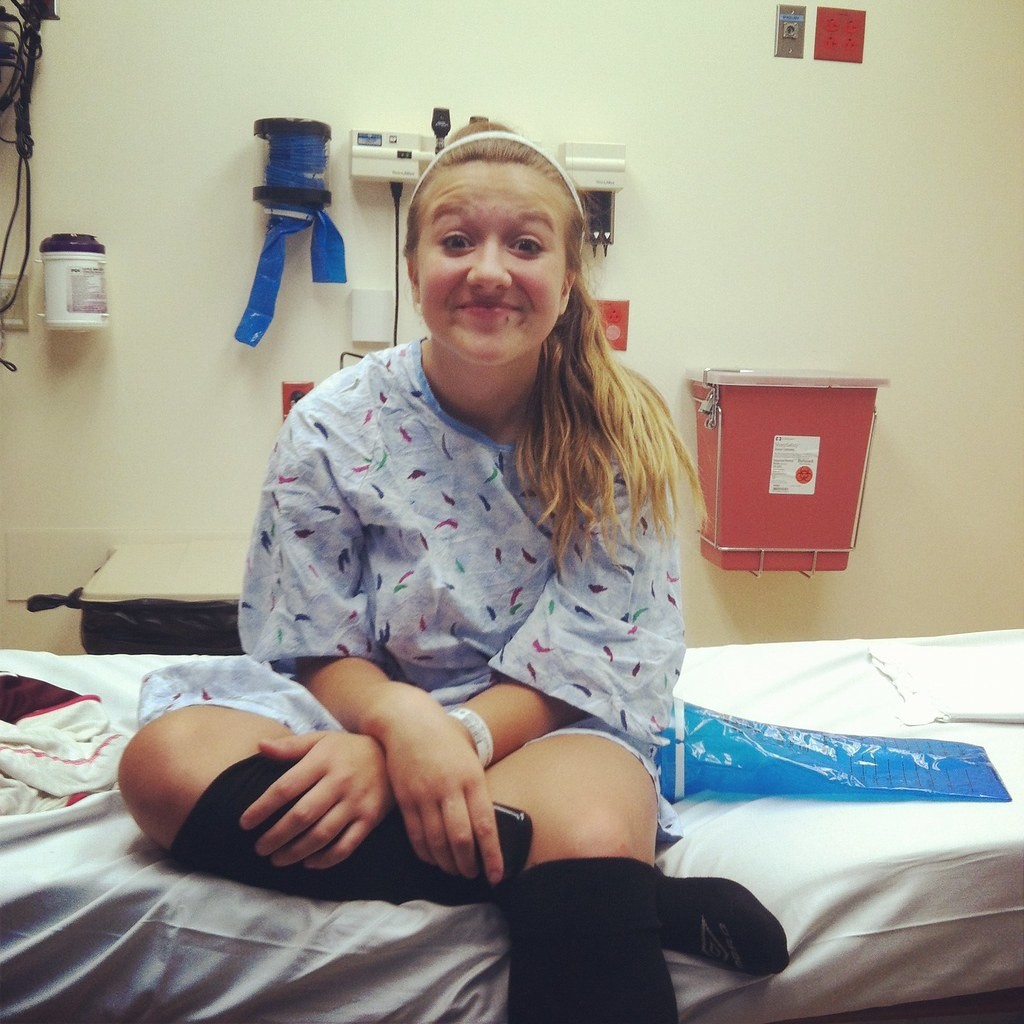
xmin=448 ymin=708 xmax=493 ymax=768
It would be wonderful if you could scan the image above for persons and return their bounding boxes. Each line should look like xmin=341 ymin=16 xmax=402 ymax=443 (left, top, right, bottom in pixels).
xmin=115 ymin=124 xmax=791 ymax=1024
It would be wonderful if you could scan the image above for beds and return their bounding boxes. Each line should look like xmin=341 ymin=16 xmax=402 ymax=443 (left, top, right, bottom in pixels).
xmin=1 ymin=628 xmax=1024 ymax=1024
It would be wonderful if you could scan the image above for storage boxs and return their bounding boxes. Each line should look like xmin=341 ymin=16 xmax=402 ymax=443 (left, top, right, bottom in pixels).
xmin=688 ymin=369 xmax=891 ymax=572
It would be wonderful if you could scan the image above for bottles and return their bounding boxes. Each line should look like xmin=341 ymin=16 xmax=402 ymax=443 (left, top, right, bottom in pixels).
xmin=40 ymin=232 xmax=109 ymax=334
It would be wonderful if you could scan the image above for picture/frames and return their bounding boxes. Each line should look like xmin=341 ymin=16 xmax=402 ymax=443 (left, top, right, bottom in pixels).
xmin=0 ymin=273 xmax=29 ymax=329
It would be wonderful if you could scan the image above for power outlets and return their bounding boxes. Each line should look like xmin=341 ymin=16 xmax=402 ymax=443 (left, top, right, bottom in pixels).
xmin=594 ymin=300 xmax=630 ymax=352
xmin=814 ymin=6 xmax=866 ymax=63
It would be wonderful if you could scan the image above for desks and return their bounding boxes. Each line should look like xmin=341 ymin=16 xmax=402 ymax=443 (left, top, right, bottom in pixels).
xmin=81 ymin=544 xmax=243 ymax=600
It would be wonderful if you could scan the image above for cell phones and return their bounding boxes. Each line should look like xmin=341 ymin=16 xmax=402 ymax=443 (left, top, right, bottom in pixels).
xmin=470 ymin=799 xmax=534 ymax=878
